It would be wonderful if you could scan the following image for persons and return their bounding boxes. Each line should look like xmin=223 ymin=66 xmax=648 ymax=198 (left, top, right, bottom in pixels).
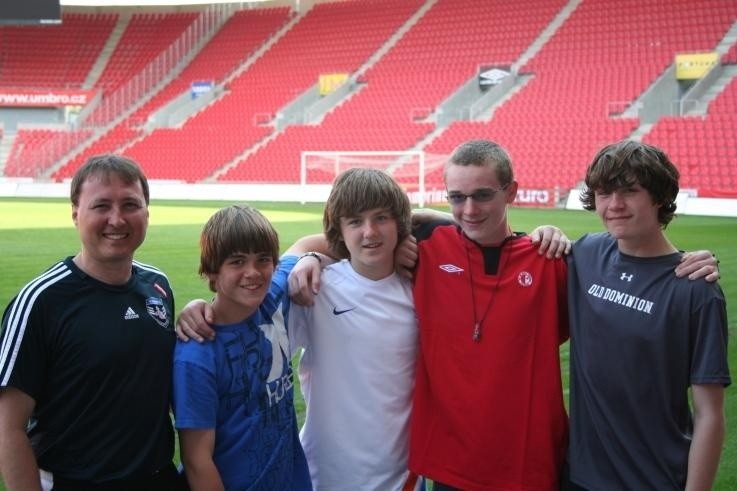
xmin=177 ymin=167 xmax=572 ymax=491
xmin=411 ymin=142 xmax=730 ymax=490
xmin=288 ymin=142 xmax=720 ymax=491
xmin=1 ymin=155 xmax=181 ymax=491
xmin=173 ymin=204 xmax=419 ymax=491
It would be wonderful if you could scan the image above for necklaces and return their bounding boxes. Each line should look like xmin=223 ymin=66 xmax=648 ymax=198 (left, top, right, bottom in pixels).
xmin=467 ymin=230 xmax=515 ymax=342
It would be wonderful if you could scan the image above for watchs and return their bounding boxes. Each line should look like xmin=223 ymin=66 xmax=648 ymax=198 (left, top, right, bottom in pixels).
xmin=298 ymin=250 xmax=322 ymax=262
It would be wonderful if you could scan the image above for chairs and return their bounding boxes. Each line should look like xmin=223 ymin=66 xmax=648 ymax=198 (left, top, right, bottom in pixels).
xmin=0 ymin=1 xmax=736 ymax=207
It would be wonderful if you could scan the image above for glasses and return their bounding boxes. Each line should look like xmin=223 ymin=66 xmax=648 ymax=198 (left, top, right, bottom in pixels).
xmin=445 ymin=183 xmax=509 ymax=206
